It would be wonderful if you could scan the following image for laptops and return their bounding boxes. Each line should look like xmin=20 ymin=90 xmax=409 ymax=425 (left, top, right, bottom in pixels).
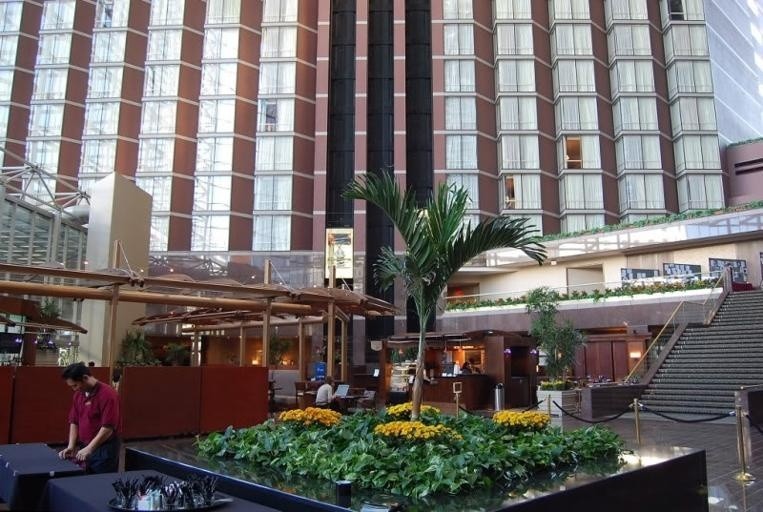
xmin=333 ymin=385 xmax=349 ymax=398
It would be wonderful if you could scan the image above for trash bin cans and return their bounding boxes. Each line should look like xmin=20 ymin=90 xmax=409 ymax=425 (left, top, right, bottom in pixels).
xmin=494 ymin=383 xmax=505 ymax=411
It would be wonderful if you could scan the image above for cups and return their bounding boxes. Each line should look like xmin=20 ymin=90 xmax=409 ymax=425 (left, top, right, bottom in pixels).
xmin=335 ymin=480 xmax=352 ymax=508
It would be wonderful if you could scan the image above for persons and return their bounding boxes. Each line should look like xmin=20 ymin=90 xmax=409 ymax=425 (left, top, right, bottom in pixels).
xmin=268 ymin=362 xmax=276 ymax=401
xmin=460 ymin=361 xmax=471 ymax=373
xmin=468 ymin=357 xmax=479 ymax=374
xmin=57 ymin=362 xmax=121 ymax=475
xmin=315 ymin=374 xmax=344 ymax=413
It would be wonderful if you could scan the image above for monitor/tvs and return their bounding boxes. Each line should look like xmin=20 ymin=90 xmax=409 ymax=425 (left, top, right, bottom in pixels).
xmin=0 ymin=331 xmax=24 ymax=354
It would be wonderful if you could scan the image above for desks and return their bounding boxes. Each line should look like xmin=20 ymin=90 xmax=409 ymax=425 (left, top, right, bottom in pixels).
xmin=335 ymin=395 xmax=369 ymax=415
xmin=0 ymin=442 xmax=282 ymax=512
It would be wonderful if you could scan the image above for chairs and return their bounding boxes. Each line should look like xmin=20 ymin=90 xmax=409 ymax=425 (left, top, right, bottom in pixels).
xmin=294 ymin=381 xmax=344 ymax=405
